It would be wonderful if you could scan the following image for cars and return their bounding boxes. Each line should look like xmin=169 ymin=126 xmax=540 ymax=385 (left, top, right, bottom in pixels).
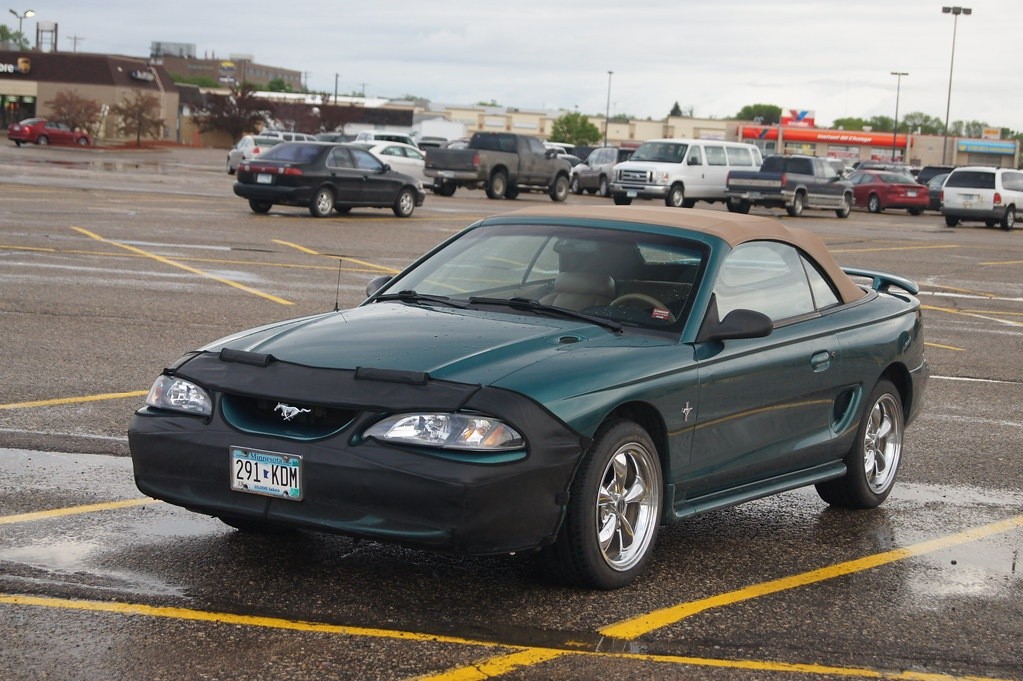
xmin=125 ymin=208 xmax=930 ymax=590
xmin=225 ymin=135 xmax=286 ymax=175
xmin=345 ymin=140 xmax=434 ymax=187
xmin=232 ymin=141 xmax=427 ymax=218
xmin=846 ymin=171 xmax=930 ymax=216
xmin=259 ymin=131 xmax=582 ymax=194
xmin=7 ymin=118 xmax=92 ymax=147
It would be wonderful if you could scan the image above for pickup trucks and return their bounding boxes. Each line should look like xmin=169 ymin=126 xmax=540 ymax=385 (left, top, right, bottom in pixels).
xmin=423 ymin=130 xmax=572 ymax=202
xmin=722 ymin=153 xmax=856 ymax=219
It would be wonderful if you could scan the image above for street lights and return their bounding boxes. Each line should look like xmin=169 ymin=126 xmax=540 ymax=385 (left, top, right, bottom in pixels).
xmin=604 ymin=71 xmax=614 ymax=148
xmin=940 ymin=5 xmax=974 ymax=164
xmin=890 ymin=72 xmax=910 ymax=162
xmin=9 ymin=8 xmax=35 ymax=51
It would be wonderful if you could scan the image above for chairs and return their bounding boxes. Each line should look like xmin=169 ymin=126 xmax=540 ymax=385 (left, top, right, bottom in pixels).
xmin=543 ymin=271 xmax=617 ymax=313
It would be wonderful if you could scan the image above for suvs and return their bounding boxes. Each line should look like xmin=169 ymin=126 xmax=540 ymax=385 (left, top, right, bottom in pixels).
xmin=821 ymin=157 xmax=954 ymax=212
xmin=939 ymin=166 xmax=1023 ymax=233
xmin=571 ymin=146 xmax=636 ymax=198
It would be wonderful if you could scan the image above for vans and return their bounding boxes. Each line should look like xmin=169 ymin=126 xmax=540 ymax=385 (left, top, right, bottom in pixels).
xmin=608 ymin=139 xmax=763 ymax=208
